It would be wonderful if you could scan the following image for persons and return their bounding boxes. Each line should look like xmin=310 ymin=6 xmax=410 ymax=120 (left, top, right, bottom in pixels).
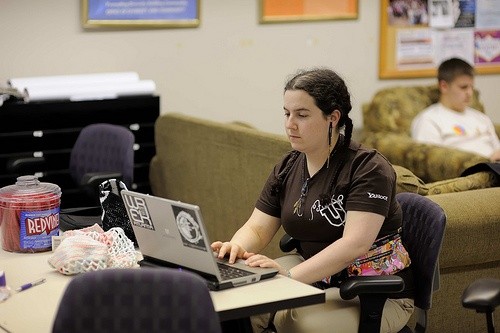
xmin=210 ymin=65 xmax=415 ymax=333
xmin=409 ymin=58 xmax=500 ymax=162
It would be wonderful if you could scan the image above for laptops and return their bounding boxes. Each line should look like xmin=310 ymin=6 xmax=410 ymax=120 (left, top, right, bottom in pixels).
xmin=121 ymin=189 xmax=280 ymax=290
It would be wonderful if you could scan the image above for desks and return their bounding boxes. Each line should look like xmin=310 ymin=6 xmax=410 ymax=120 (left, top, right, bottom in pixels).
xmin=0 ymin=235 xmax=325 ymax=333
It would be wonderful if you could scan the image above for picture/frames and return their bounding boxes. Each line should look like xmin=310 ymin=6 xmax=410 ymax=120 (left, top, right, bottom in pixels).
xmin=379 ymin=0 xmax=500 ymax=79
xmin=257 ymin=0 xmax=358 ymax=23
xmin=80 ymin=0 xmax=201 ymax=31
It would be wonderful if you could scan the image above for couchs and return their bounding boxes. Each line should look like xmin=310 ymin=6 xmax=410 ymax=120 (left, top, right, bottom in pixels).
xmin=148 ymin=85 xmax=500 ymax=332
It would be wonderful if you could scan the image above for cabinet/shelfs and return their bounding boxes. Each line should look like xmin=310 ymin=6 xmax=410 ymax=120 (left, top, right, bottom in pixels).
xmin=0 ymin=92 xmax=160 ymax=214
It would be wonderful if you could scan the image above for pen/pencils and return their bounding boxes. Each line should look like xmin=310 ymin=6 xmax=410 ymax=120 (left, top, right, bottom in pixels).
xmin=16 ymin=277 xmax=45 ymax=292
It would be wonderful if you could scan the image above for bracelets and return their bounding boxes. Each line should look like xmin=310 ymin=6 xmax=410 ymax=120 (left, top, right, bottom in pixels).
xmin=287 ymin=269 xmax=291 ymax=278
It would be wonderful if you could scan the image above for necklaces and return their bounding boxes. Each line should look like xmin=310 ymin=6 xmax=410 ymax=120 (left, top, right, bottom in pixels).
xmin=292 ymin=184 xmax=307 ymax=217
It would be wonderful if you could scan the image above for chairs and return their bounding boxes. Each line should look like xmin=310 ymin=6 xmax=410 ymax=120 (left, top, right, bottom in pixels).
xmin=280 ymin=193 xmax=446 ymax=333
xmin=52 ymin=268 xmax=222 ymax=333
xmin=10 ymin=123 xmax=134 ymax=232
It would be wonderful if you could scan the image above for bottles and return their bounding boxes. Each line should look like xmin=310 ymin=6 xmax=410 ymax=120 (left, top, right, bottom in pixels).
xmin=0 ymin=176 xmax=62 ymax=254
